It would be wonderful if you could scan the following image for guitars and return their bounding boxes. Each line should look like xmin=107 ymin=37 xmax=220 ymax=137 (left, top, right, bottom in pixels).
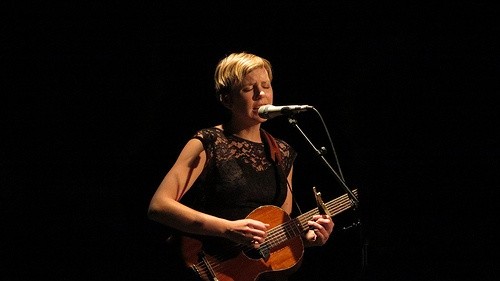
xmin=180 ymin=188 xmax=359 ymax=281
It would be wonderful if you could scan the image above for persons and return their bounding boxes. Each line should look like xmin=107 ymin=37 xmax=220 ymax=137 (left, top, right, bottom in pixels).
xmin=147 ymin=52 xmax=334 ymax=281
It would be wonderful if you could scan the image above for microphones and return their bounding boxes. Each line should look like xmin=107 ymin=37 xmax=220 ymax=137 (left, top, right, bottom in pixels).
xmin=258 ymin=104 xmax=313 ymax=118
